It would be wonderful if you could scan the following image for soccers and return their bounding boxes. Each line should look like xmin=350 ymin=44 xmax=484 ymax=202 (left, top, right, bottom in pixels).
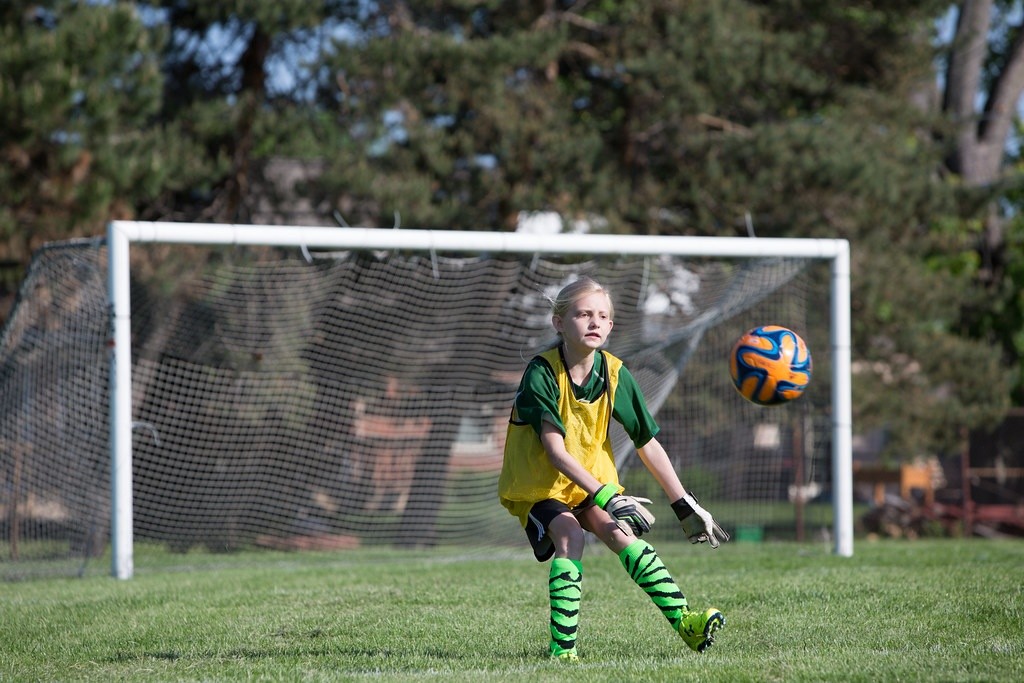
xmin=728 ymin=325 xmax=813 ymax=407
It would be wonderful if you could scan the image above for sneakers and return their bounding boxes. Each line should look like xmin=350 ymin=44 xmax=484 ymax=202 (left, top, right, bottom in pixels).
xmin=547 ymin=648 xmax=579 ymax=665
xmin=677 ymin=606 xmax=725 ymax=652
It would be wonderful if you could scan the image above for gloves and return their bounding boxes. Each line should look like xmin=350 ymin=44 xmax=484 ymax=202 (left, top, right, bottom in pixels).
xmin=593 ymin=483 xmax=657 ymax=537
xmin=670 ymin=490 xmax=730 ymax=549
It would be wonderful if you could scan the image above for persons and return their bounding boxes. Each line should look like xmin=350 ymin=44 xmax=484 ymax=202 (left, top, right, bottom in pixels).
xmin=497 ymin=276 xmax=731 ymax=662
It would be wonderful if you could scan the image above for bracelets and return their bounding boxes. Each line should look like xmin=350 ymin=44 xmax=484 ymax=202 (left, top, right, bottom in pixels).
xmin=594 ymin=482 xmax=618 ymax=510
